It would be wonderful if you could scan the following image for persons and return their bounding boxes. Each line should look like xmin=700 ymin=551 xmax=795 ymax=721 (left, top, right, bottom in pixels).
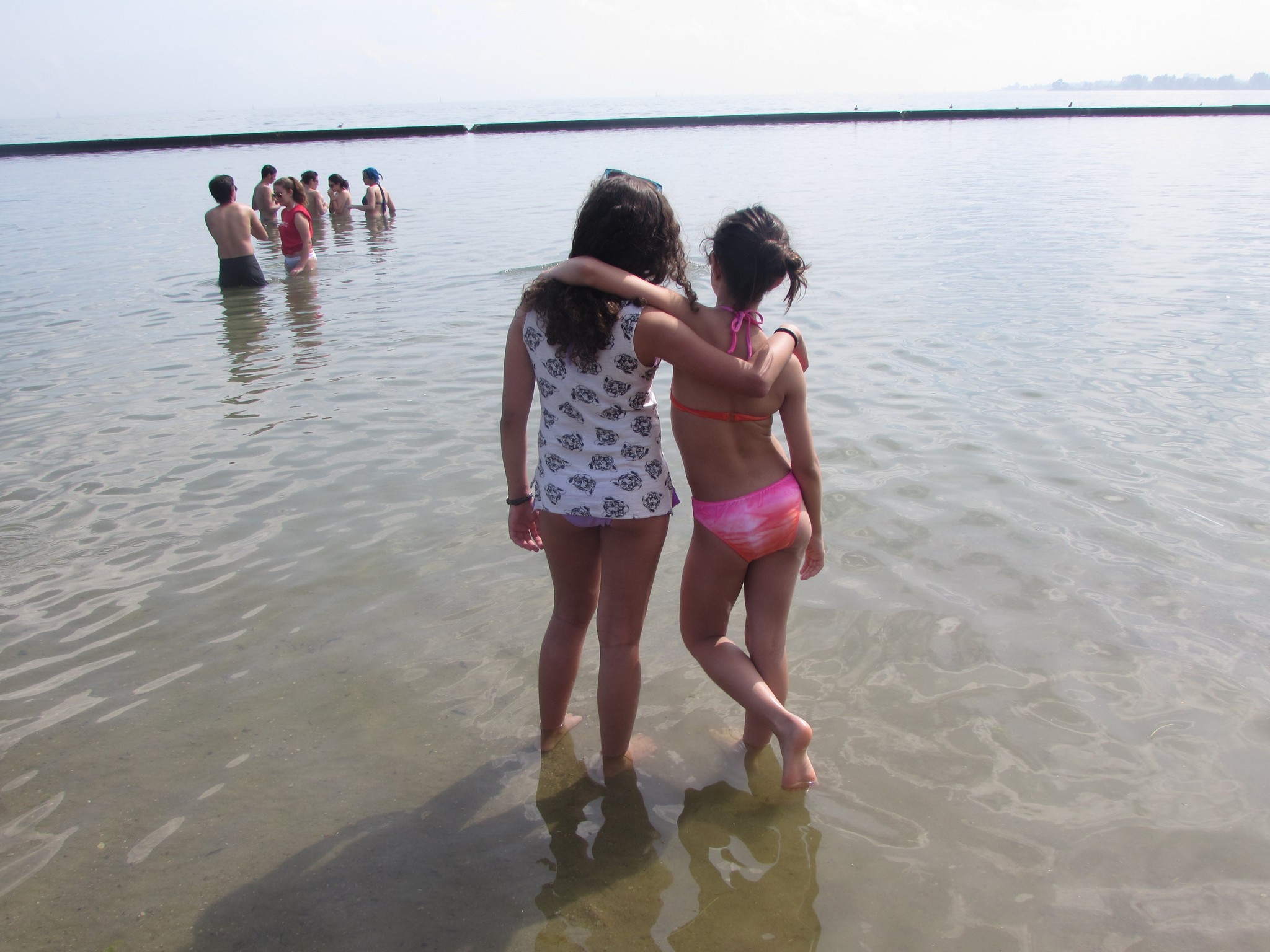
xmin=499 ymin=168 xmax=808 ymax=756
xmin=205 ymin=175 xmax=269 ymax=284
xmin=272 ymin=176 xmax=317 ymax=271
xmin=534 ymin=206 xmax=825 ymax=787
xmin=300 ymin=171 xmax=329 ymax=218
xmin=347 ymin=167 xmax=396 ymax=216
xmin=252 ymin=165 xmax=282 ymax=219
xmin=327 ymin=174 xmax=352 ymax=216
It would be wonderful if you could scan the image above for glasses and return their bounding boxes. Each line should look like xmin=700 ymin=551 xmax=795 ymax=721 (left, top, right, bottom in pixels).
xmin=310 ymin=180 xmax=318 ymax=184
xmin=598 ymin=168 xmax=663 ymax=194
xmin=363 ymin=176 xmax=369 ymax=178
xmin=272 ymin=191 xmax=291 ymax=197
xmin=233 ymin=184 xmax=237 ymax=192
xmin=328 ymin=183 xmax=338 ymax=189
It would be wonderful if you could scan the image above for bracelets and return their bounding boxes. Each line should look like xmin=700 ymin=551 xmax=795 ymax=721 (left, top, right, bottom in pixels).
xmin=506 ymin=491 xmax=533 ymax=505
xmin=773 ymin=328 xmax=798 ymax=348
xmin=332 ymin=199 xmax=337 ymax=201
